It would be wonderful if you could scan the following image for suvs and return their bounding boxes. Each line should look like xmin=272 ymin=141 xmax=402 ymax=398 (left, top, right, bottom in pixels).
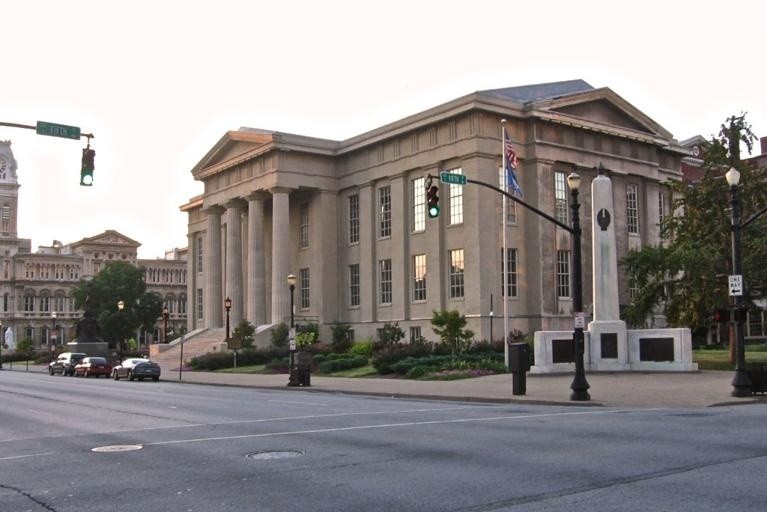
xmin=48 ymin=351 xmax=88 ymax=376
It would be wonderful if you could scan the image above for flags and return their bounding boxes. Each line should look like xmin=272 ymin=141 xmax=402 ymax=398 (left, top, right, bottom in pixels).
xmin=502 ymin=126 xmax=522 ymax=198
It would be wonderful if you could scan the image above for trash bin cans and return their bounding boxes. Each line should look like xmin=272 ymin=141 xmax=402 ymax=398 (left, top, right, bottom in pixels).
xmin=287 ymin=351 xmax=312 ymax=386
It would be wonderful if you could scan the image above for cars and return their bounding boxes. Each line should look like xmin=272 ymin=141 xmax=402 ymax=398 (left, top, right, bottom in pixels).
xmin=112 ymin=357 xmax=162 ymax=382
xmin=73 ymin=355 xmax=111 ymax=378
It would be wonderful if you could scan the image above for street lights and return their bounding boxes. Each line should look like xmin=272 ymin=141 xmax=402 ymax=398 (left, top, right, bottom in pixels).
xmin=285 ymin=273 xmax=296 ymax=386
xmin=567 ymin=171 xmax=592 ymax=400
xmin=49 ymin=310 xmax=58 ymax=361
xmin=723 ymin=168 xmax=754 ymax=398
xmin=224 ymin=296 xmax=231 ymax=342
xmin=163 ymin=304 xmax=169 ymax=343
xmin=117 ymin=300 xmax=126 ymax=359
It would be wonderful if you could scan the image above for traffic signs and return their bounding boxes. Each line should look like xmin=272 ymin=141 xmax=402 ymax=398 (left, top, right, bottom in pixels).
xmin=440 ymin=172 xmax=467 ymax=185
xmin=729 ymin=275 xmax=742 ymax=295
xmin=35 ymin=121 xmax=81 ymax=140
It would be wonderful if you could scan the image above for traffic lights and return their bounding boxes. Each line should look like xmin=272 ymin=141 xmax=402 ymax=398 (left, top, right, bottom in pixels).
xmin=79 ymin=148 xmax=96 ymax=186
xmin=710 ymin=305 xmax=729 ymax=323
xmin=427 ymin=186 xmax=440 ymax=218
